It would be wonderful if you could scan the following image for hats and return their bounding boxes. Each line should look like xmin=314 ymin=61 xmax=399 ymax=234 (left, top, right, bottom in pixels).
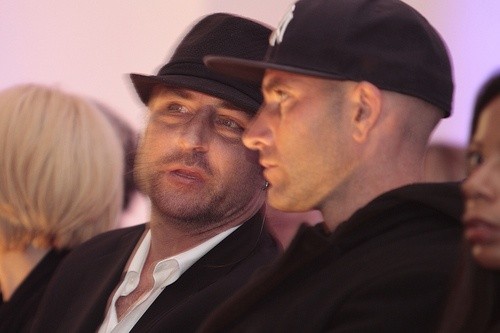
xmin=127 ymin=11 xmax=276 ymax=114
xmin=202 ymin=0 xmax=455 ymax=120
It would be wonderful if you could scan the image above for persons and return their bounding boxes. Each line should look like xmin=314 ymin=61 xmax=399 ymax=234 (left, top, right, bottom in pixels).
xmin=1 ymin=13 xmax=287 ymax=333
xmin=197 ymin=0 xmax=471 ymax=333
xmin=445 ymin=66 xmax=500 ymax=333
xmin=1 ymin=84 xmax=126 ymax=311
